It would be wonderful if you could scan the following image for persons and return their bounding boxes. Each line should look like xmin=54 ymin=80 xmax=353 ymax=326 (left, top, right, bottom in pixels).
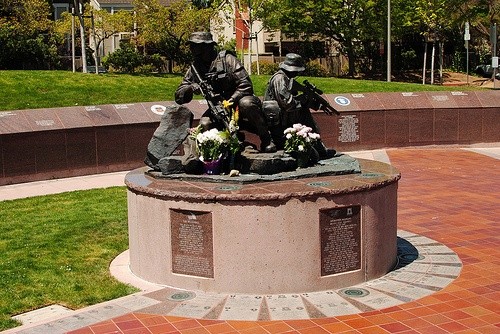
xmin=174 ymin=32 xmax=268 ymax=153
xmin=263 ymin=52 xmax=336 ymax=158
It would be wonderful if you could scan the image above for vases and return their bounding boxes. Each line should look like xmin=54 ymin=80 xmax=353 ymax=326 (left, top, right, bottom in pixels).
xmin=199 ymin=155 xmax=222 ymax=174
xmin=297 ymin=152 xmax=319 ymax=168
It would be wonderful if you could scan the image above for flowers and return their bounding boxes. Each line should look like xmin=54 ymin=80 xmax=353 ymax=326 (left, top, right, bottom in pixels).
xmin=282 ymin=121 xmax=319 ymax=154
xmin=187 ymin=126 xmax=231 ymax=158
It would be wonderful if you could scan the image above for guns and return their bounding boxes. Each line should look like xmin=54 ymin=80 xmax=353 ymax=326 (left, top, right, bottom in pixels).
xmin=287 ymin=78 xmax=340 ymax=115
xmin=192 ymin=65 xmax=229 ymax=126
